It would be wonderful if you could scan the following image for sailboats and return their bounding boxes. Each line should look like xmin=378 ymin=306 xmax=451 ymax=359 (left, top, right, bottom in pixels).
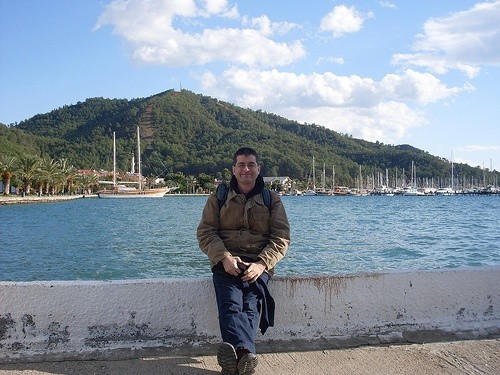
xmin=326 ymin=163 xmax=350 ymax=196
xmin=404 ymin=165 xmax=424 ymax=196
xmin=442 ymin=178 xmax=455 ymax=196
xmin=347 ymin=164 xmax=369 ymax=197
xmin=304 ymin=155 xmax=318 ymax=197
xmin=316 ymin=162 xmax=333 ymax=195
xmin=97 ymin=126 xmax=179 ymax=198
xmin=364 ymin=158 xmax=500 ymax=196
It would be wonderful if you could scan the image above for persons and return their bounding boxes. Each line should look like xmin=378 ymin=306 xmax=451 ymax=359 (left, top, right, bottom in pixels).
xmin=196 ymin=147 xmax=292 ymax=375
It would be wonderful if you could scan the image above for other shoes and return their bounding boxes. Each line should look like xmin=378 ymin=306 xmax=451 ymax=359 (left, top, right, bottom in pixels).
xmin=217 ymin=342 xmax=238 ymax=375
xmin=237 ymin=352 xmax=259 ymax=375
